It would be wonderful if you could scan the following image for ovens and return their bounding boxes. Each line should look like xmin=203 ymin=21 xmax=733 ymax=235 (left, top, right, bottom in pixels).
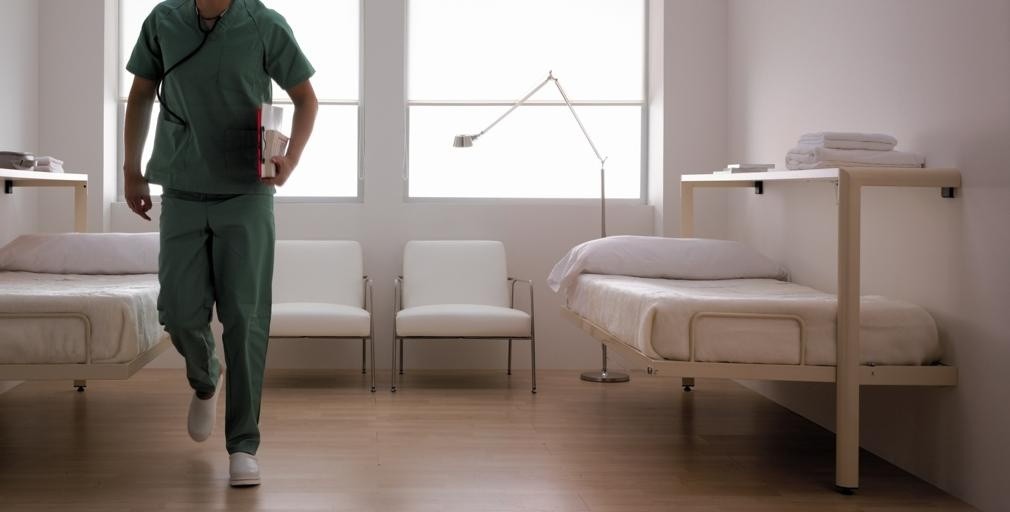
xmin=0 ymin=167 xmax=173 ymax=391
xmin=553 ymin=166 xmax=961 ymax=496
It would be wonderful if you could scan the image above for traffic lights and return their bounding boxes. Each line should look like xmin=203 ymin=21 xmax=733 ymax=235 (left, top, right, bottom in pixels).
xmin=1 ymin=229 xmax=163 ymax=277
xmin=546 ymin=233 xmax=792 ymax=294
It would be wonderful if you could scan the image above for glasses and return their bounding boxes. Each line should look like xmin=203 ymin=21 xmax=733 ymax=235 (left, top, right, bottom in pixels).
xmin=449 ymin=71 xmax=631 ymax=384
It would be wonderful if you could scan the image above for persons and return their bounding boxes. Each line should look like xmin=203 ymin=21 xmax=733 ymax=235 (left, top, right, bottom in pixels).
xmin=123 ymin=0 xmax=318 ymax=487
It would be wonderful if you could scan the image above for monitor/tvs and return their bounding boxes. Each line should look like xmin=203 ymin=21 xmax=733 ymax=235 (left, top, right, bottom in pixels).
xmin=264 ymin=130 xmax=289 ymax=178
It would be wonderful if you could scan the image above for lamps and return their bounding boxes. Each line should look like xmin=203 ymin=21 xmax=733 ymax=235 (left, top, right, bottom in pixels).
xmin=390 ymin=238 xmax=538 ymax=397
xmin=268 ymin=238 xmax=376 ymax=395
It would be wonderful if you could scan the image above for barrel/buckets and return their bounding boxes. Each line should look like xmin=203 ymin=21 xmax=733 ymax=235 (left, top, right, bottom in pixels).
xmin=187 ymin=373 xmax=224 ymax=443
xmin=230 ymin=452 xmax=260 ymax=487
